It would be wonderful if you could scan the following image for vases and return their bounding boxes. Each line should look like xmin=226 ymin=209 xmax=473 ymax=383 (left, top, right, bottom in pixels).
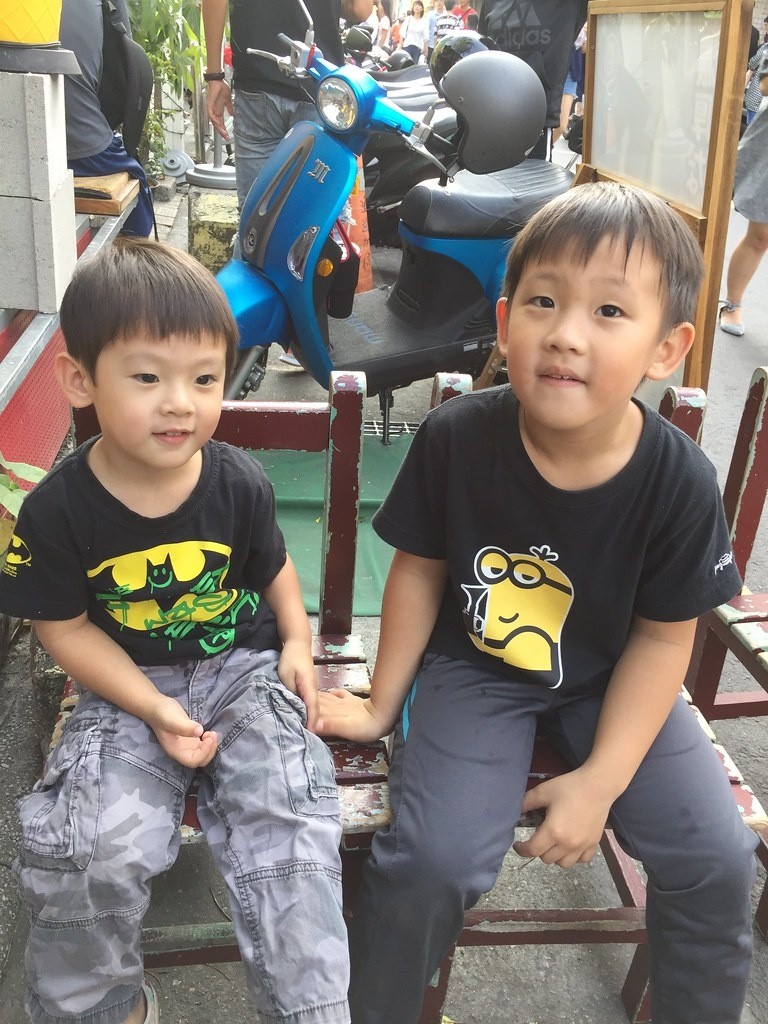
xmin=0 ymin=0 xmax=64 ymax=49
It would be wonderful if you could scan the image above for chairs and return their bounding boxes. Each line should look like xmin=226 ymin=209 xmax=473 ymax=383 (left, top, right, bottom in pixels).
xmin=40 ymin=371 xmax=391 ymax=970
xmin=417 ymin=371 xmax=768 ymax=1024
xmin=681 ymin=367 xmax=768 ymax=726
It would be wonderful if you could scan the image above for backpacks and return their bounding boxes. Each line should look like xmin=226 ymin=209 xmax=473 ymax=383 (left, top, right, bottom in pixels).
xmin=101 ymin=1 xmax=153 ymax=161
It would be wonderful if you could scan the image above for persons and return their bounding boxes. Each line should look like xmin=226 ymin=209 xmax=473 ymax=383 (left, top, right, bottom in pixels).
xmin=1 ymin=239 xmax=353 ymax=1024
xmin=551 ymin=16 xmax=588 ymax=147
xmin=312 ymin=183 xmax=757 ymax=1024
xmin=716 ymin=15 xmax=768 ymax=338
xmin=476 ymin=0 xmax=588 ymax=160
xmin=202 ymin=0 xmax=374 ymax=223
xmin=339 ymin=0 xmax=480 ymax=72
xmin=57 ymin=0 xmax=157 ymax=239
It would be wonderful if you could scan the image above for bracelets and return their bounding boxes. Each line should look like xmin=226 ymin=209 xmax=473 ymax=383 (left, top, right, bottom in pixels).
xmin=204 ymin=72 xmax=225 ymax=81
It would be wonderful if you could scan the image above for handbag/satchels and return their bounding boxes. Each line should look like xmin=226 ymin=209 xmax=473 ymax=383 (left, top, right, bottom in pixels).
xmin=325 ymin=221 xmax=359 ymax=321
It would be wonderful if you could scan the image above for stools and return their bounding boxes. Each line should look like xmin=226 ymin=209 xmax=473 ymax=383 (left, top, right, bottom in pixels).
xmin=75 ymin=172 xmax=141 ymax=217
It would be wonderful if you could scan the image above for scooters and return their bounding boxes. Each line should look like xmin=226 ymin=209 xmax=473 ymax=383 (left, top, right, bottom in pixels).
xmin=211 ymin=0 xmax=577 ymax=444
xmin=220 ymin=24 xmax=501 ymax=223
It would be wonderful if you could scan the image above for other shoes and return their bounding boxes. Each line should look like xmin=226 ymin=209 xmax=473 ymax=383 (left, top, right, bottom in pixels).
xmin=281 ymin=353 xmax=301 ymax=366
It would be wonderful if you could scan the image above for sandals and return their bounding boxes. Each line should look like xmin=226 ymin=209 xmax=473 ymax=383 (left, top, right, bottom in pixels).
xmin=719 ymin=301 xmax=745 ymax=337
